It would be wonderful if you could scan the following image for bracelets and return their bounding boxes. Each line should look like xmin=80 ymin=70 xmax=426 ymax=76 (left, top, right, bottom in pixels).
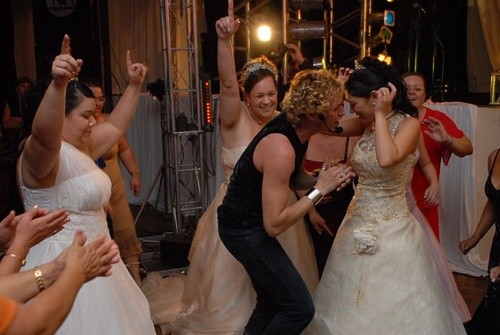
xmin=4 ymin=252 xmax=27 ymax=267
xmin=33 ymin=265 xmax=46 ymax=292
xmin=304 ymin=187 xmax=323 ymax=207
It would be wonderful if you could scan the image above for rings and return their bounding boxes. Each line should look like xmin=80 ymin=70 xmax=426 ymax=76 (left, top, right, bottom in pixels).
xmin=339 ymin=170 xmax=348 ymax=178
xmin=321 ymin=167 xmax=327 ymax=170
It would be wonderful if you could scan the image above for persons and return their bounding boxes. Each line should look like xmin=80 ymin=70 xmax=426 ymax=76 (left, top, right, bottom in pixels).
xmin=0 ymin=0 xmax=500 ymax=335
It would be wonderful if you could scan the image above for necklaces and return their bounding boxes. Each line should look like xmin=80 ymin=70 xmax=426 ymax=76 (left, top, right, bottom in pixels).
xmin=385 ymin=109 xmax=396 ymax=121
xmin=247 ymin=110 xmax=262 ymax=126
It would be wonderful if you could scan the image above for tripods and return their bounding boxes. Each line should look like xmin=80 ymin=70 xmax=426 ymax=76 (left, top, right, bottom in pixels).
xmin=134 ymin=101 xmax=197 ymax=226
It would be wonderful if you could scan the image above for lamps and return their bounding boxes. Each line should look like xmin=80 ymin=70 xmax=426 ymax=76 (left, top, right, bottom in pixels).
xmin=368 ymin=10 xmax=395 ymax=26
xmin=367 ymin=26 xmax=393 ymax=48
xmin=313 ymin=57 xmax=327 ymax=70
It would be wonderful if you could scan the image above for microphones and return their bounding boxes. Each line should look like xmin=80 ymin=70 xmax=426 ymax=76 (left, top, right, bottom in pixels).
xmin=318 ymin=113 xmax=343 ymax=134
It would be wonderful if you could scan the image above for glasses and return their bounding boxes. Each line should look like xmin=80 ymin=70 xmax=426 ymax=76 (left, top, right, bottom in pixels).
xmin=95 ymin=98 xmax=105 ymax=103
